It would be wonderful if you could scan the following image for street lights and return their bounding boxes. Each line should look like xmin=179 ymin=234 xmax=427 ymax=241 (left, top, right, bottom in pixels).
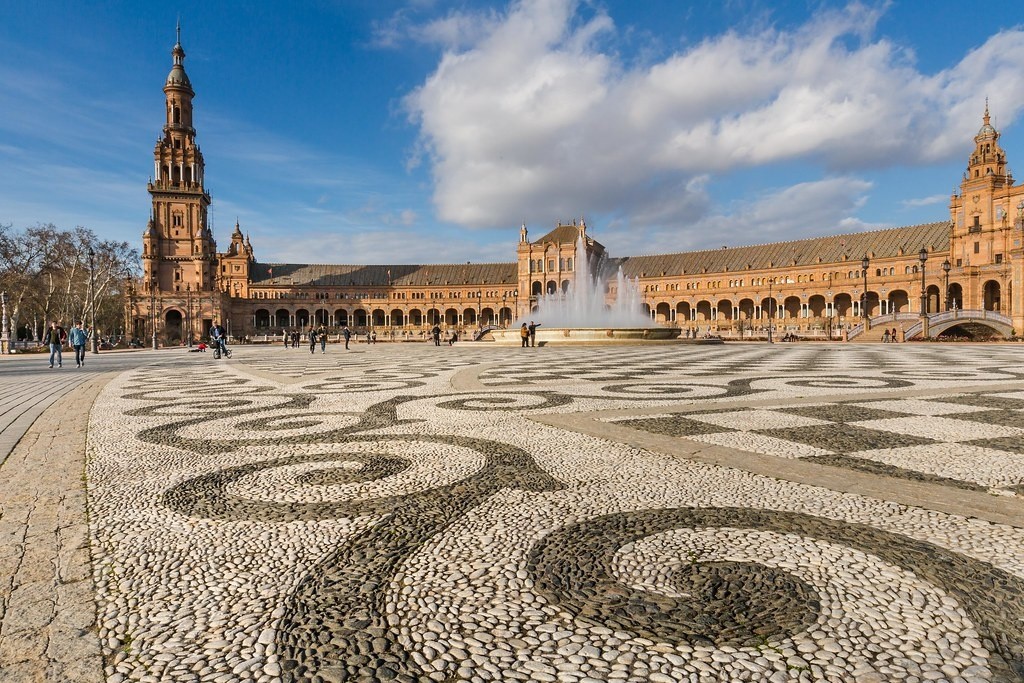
xmin=150 ymin=273 xmax=158 ymax=350
xmin=767 ymin=278 xmax=774 ymax=344
xmin=185 ymin=282 xmax=192 ymax=347
xmin=860 ymin=254 xmax=870 ymax=318
xmin=502 ymin=295 xmax=506 ymax=329
xmin=87 ymin=247 xmax=98 ymax=354
xmin=514 ymin=289 xmax=518 ymax=320
xmin=918 ymin=244 xmax=928 ymax=316
xmin=477 ymin=289 xmax=481 ymax=328
xmin=942 ymin=256 xmax=952 ymax=311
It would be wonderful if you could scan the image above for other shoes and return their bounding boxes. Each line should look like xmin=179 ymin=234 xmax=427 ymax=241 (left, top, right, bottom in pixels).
xmin=309 ymin=351 xmax=314 ymax=354
xmin=76 ymin=365 xmax=80 ymax=368
xmin=57 ymin=365 xmax=62 ymax=368
xmin=80 ymin=361 xmax=84 ymax=367
xmin=522 ymin=346 xmax=525 ymax=347
xmin=216 ymin=351 xmax=220 ymax=355
xmin=49 ymin=365 xmax=53 ymax=368
xmin=322 ymin=351 xmax=326 ymax=355
xmin=225 ymin=351 xmax=228 ymax=356
xmin=532 ymin=345 xmax=535 ymax=347
xmin=527 ymin=346 xmax=530 ymax=347
xmin=345 ymin=348 xmax=350 ymax=350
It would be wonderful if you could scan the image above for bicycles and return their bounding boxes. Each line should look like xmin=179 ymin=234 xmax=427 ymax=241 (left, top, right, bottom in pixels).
xmin=208 ymin=337 xmax=233 ymax=359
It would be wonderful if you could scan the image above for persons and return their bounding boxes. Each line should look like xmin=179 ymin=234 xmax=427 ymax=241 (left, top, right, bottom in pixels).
xmin=366 ymin=330 xmax=377 ymax=344
xmin=521 ymin=323 xmax=530 ymax=348
xmin=198 ymin=342 xmax=208 ymax=352
xmin=68 ymin=321 xmax=88 ymax=368
xmin=291 ymin=330 xmax=300 ymax=348
xmin=97 ymin=335 xmax=115 ymax=350
xmin=448 ymin=331 xmax=458 ymax=346
xmin=282 ymin=329 xmax=288 ymax=350
xmin=528 ymin=321 xmax=541 ymax=347
xmin=685 ymin=327 xmax=698 ymax=339
xmin=40 ymin=321 xmax=67 ymax=368
xmin=343 ymin=325 xmax=351 ymax=350
xmin=317 ymin=323 xmax=328 ymax=354
xmin=308 ymin=325 xmax=318 ymax=354
xmin=431 ymin=323 xmax=441 ymax=346
xmin=782 ymin=333 xmax=800 ymax=342
xmin=882 ymin=328 xmax=899 ymax=343
xmin=209 ymin=320 xmax=232 ymax=358
xmin=130 ymin=338 xmax=145 ymax=348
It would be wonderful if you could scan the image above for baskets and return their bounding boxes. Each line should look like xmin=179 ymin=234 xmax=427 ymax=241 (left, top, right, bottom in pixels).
xmin=209 ymin=340 xmax=219 ymax=348
